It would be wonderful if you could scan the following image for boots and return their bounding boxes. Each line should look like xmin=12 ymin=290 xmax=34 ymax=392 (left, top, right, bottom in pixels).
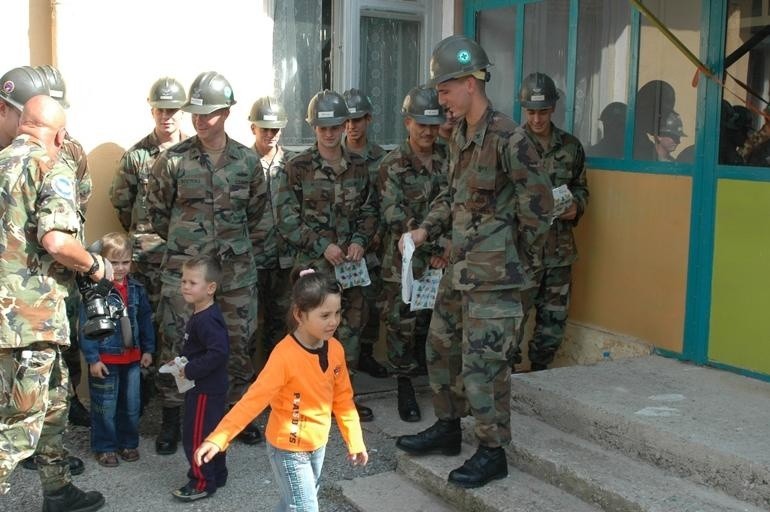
xmin=68 ymin=393 xmax=92 ymax=428
xmin=155 ymin=406 xmax=181 ymax=455
xmin=225 ymin=402 xmax=262 ymax=445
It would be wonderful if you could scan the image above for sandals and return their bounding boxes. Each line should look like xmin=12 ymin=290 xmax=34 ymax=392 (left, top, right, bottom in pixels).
xmin=94 ymin=450 xmax=119 ymax=467
xmin=117 ymin=447 xmax=140 ymax=462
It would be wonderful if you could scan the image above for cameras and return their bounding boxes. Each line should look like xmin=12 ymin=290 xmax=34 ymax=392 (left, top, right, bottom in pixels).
xmin=71 ymin=267 xmax=119 ymax=342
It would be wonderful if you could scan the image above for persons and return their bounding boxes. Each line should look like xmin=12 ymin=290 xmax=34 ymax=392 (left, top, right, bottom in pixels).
xmin=586 ymin=80 xmax=769 ymax=169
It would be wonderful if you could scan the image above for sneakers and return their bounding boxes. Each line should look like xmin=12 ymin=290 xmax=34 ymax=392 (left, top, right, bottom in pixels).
xmin=172 ymin=483 xmax=209 ymax=502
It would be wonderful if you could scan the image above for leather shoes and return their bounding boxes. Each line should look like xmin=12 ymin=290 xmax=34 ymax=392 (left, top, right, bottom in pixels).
xmin=396 ymin=375 xmax=422 ymax=422
xmin=332 ymin=403 xmax=374 ymax=422
xmin=22 ymin=454 xmax=85 ymax=475
xmin=39 ymin=481 xmax=106 ymax=512
xmin=446 ymin=445 xmax=509 ymax=488
xmin=356 ymin=352 xmax=389 ymax=378
xmin=394 ymin=417 xmax=462 ymax=456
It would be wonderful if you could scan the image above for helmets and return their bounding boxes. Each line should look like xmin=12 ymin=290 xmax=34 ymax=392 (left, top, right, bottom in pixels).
xmin=421 ymin=33 xmax=496 ymax=89
xmin=33 ymin=63 xmax=70 ymax=109
xmin=400 ymin=83 xmax=447 ymax=125
xmin=597 ymin=78 xmax=760 ymax=142
xmin=179 ymin=71 xmax=237 ymax=115
xmin=0 ymin=66 xmax=50 ymax=112
xmin=146 ymin=77 xmax=187 ymax=109
xmin=518 ymin=72 xmax=558 ymax=110
xmin=305 ymin=89 xmax=351 ymax=128
xmin=248 ymin=95 xmax=289 ymax=129
xmin=341 ymin=87 xmax=373 ymax=120
